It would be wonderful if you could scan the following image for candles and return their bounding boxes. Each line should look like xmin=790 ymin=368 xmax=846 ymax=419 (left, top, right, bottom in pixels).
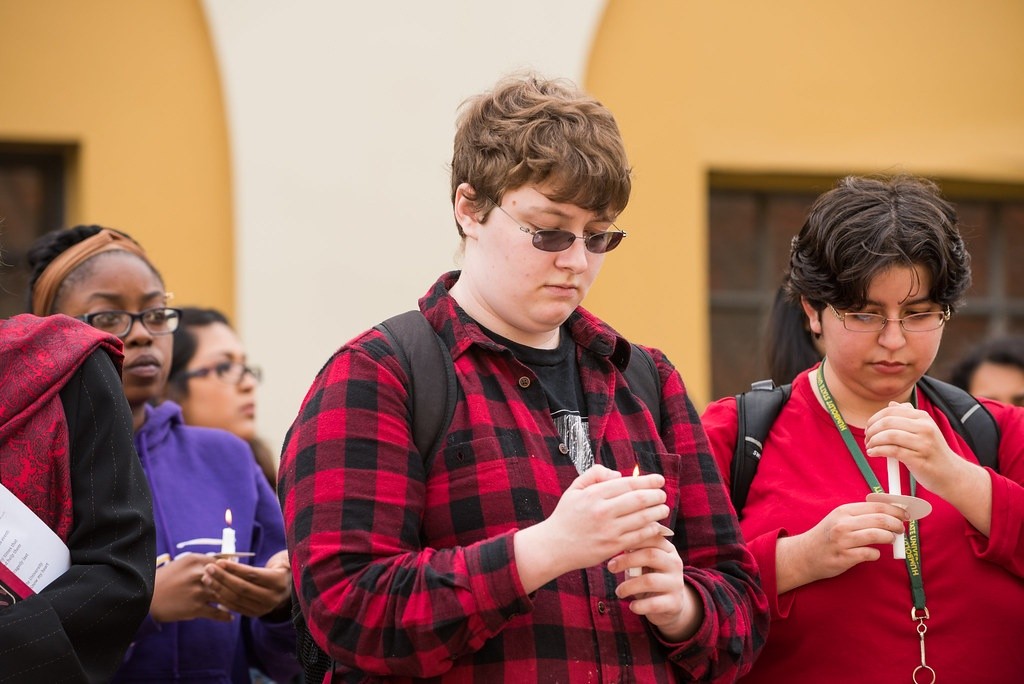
xmin=629 ymin=465 xmax=644 ymax=580
xmin=217 ymin=508 xmax=236 ymax=614
xmin=888 ymin=456 xmax=906 ymax=560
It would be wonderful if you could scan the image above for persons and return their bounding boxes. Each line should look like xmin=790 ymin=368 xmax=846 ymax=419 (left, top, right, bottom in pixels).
xmin=18 ymin=224 xmax=306 ymax=684
xmin=162 ymin=307 xmax=278 ymax=492
xmin=699 ymin=176 xmax=1024 ymax=684
xmin=760 ymin=273 xmax=825 ymax=386
xmin=951 ymin=341 xmax=1024 ymax=408
xmin=0 ymin=314 xmax=156 ymax=684
xmin=277 ymin=72 xmax=771 ymax=684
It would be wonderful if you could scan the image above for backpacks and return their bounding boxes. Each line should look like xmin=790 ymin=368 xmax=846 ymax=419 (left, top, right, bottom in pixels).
xmin=288 ymin=305 xmax=667 ymax=684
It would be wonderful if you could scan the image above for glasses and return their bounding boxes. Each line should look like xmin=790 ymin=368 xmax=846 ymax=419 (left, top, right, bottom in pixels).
xmin=180 ymin=360 xmax=265 ymax=386
xmin=72 ymin=306 xmax=184 ymax=339
xmin=475 ymin=188 xmax=628 ymax=255
xmin=821 ymin=299 xmax=951 ymax=332
